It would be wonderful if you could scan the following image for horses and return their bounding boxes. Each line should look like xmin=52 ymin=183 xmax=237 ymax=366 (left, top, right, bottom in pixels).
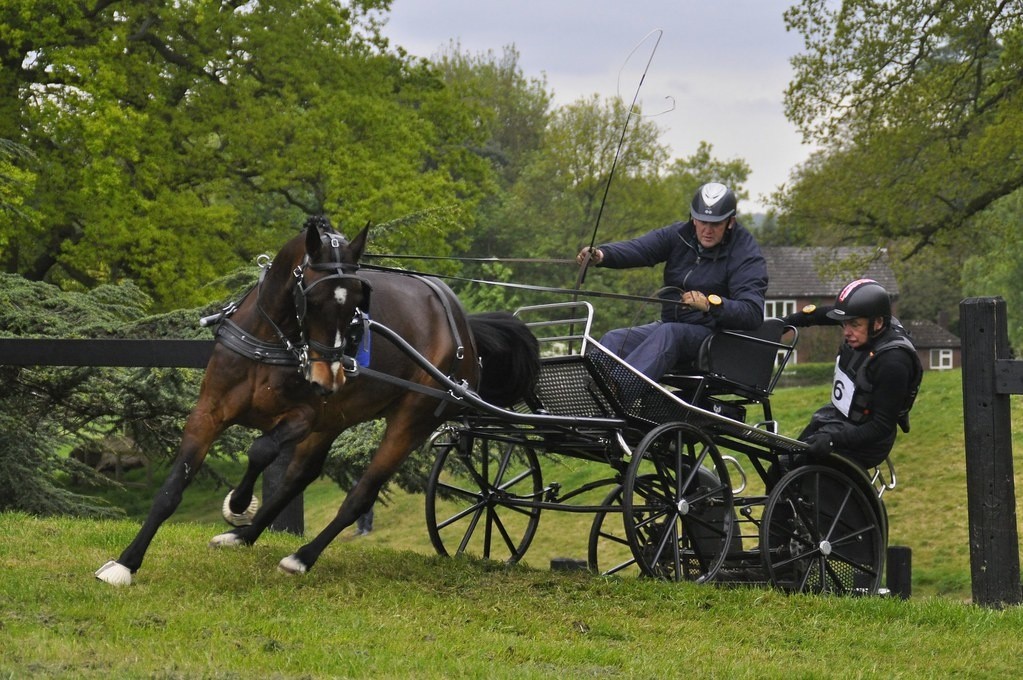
xmin=95 ymin=219 xmax=542 ymax=587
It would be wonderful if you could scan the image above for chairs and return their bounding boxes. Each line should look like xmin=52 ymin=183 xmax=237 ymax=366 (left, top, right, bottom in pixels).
xmin=661 ymin=317 xmax=797 ymax=451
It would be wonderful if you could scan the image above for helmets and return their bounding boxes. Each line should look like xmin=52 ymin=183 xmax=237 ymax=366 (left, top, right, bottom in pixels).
xmin=826 ymin=279 xmax=892 ymax=340
xmin=691 ymin=182 xmax=736 ymax=223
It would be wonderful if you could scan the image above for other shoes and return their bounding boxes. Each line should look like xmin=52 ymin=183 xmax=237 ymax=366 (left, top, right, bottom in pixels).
xmin=715 ymin=566 xmax=768 ymax=582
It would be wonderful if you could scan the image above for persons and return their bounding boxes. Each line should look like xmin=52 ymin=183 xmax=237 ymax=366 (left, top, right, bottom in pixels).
xmin=576 ymin=183 xmax=769 ymax=403
xmin=751 ymin=279 xmax=924 ymax=550
xmin=354 ymin=505 xmax=374 ymax=536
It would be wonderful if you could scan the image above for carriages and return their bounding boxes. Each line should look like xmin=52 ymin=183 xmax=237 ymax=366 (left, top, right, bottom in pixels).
xmin=95 ymin=221 xmax=898 ymax=598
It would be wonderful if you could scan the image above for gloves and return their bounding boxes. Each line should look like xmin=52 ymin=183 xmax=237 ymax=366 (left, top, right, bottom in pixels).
xmin=576 ymin=247 xmax=600 ymax=267
xmin=681 ymin=291 xmax=710 ymax=313
xmin=804 ymin=433 xmax=835 ymax=461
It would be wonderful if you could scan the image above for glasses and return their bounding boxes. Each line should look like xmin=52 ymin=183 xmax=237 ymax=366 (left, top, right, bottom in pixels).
xmin=840 ymin=318 xmax=871 ymax=330
